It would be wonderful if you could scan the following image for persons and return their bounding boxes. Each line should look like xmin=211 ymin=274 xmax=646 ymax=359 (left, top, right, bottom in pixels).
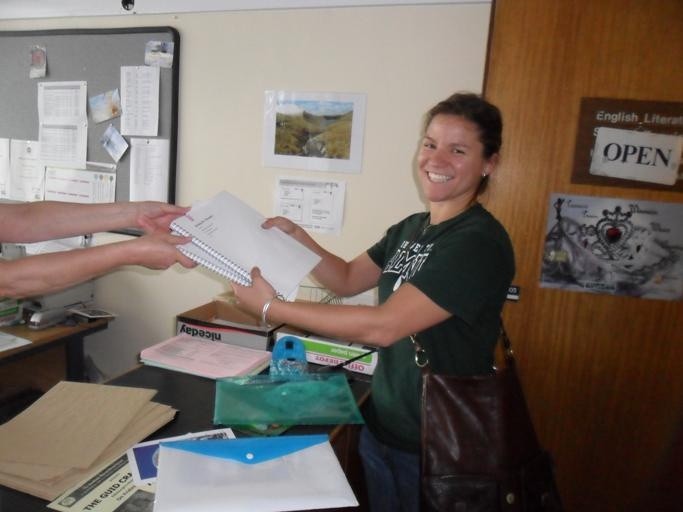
xmin=230 ymin=91 xmax=518 ymax=512
xmin=0 ymin=199 xmax=198 ymax=296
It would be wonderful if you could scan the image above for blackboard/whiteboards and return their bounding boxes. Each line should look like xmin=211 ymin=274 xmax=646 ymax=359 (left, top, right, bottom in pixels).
xmin=0 ymin=26 xmax=180 ymax=236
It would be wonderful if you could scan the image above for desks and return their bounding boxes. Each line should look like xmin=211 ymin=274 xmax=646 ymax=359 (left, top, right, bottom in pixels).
xmin=1 ymin=312 xmax=109 ymax=401
xmin=0 ymin=364 xmax=372 ymax=512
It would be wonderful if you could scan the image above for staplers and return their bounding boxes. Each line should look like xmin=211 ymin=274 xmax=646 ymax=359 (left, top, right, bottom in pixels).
xmin=29 ymin=307 xmax=67 ymax=331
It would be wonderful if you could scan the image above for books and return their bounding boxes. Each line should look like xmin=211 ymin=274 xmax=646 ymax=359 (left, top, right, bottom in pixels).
xmin=169 ymin=189 xmax=322 ymax=299
xmin=168 ymin=230 xmax=300 ymax=302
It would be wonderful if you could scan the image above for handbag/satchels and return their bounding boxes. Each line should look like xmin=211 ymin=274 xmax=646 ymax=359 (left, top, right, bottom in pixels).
xmin=420 ymin=365 xmax=560 ymax=511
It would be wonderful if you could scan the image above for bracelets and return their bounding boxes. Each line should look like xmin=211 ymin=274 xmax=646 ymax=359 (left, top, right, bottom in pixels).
xmin=261 ymin=294 xmax=285 ymax=329
